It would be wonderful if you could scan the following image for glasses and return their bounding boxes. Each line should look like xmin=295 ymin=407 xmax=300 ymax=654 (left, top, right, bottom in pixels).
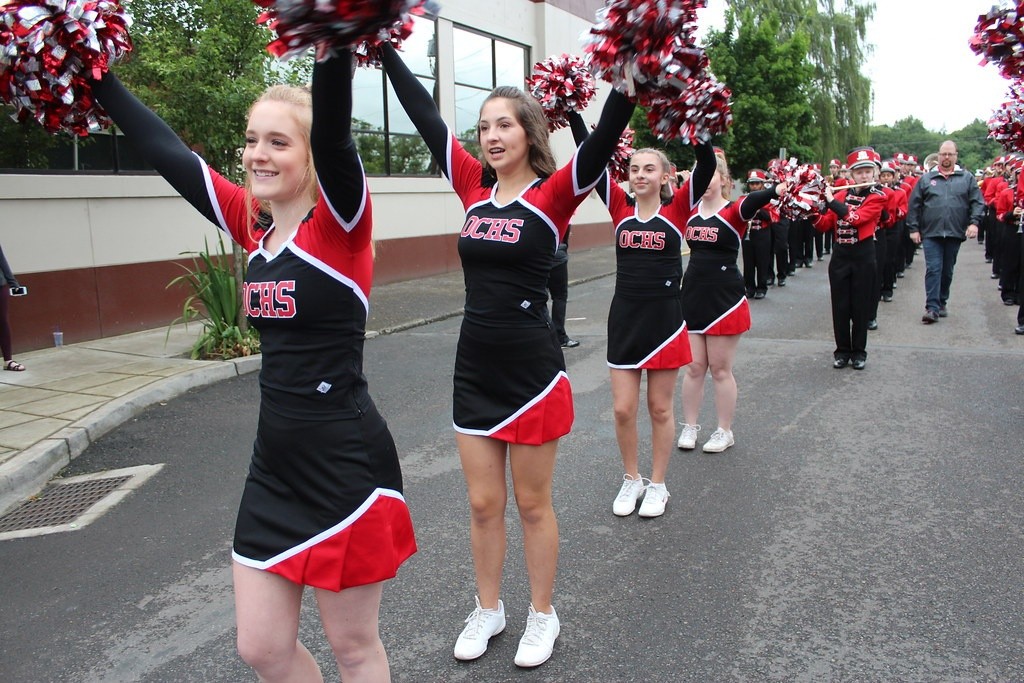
xmin=938 ymin=152 xmax=958 ymax=156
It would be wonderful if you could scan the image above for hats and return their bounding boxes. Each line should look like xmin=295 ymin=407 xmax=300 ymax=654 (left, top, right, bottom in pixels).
xmin=746 ymin=169 xmax=767 ymax=183
xmin=847 ymin=146 xmax=925 ymax=173
xmin=829 ymin=160 xmax=841 ymax=167
xmin=975 ymin=154 xmax=1024 ymax=177
xmin=840 ymin=165 xmax=848 ymax=172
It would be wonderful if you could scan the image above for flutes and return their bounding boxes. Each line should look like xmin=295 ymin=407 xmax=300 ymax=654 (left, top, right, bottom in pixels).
xmin=744 ymin=219 xmax=752 ymax=241
xmin=1018 ymin=208 xmax=1023 ymax=235
xmin=826 ymin=180 xmax=879 ymax=193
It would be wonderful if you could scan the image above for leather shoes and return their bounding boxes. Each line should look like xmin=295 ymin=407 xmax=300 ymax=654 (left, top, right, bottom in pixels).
xmin=868 ymin=320 xmax=877 ymax=330
xmin=852 ymin=360 xmax=865 ymax=369
xmin=745 ymin=289 xmax=765 ymax=299
xmin=834 ymin=358 xmax=849 ymax=369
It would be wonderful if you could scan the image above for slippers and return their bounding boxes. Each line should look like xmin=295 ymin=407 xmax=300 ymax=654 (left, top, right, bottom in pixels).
xmin=3 ymin=360 xmax=26 ymax=371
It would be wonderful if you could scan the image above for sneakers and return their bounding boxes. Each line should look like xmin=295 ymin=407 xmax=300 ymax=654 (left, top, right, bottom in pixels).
xmin=453 ymin=595 xmax=506 ymax=661
xmin=677 ymin=422 xmax=701 ymax=449
xmin=514 ymin=603 xmax=560 ymax=667
xmin=702 ymin=427 xmax=735 ymax=453
xmin=612 ymin=473 xmax=671 ymax=518
xmin=922 ymin=305 xmax=948 ymax=322
xmin=560 ymin=339 xmax=580 ymax=348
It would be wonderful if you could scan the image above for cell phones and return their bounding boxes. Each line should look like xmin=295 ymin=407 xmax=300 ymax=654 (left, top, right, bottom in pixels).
xmin=9 ymin=286 xmax=28 ymax=296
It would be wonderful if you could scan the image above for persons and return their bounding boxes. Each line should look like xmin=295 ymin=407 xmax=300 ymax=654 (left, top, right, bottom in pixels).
xmin=0 ymin=247 xmax=26 ymax=371
xmin=739 ymin=139 xmax=1024 ymax=369
xmin=567 ymin=108 xmax=717 ymax=517
xmin=81 ymin=39 xmax=417 ymax=683
xmin=378 ymin=42 xmax=639 ymax=666
xmin=674 ymin=146 xmax=789 ymax=453
xmin=547 ymin=225 xmax=580 ymax=348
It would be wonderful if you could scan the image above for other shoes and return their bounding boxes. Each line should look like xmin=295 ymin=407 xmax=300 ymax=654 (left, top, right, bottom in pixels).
xmin=881 ymin=242 xmax=923 ymax=301
xmin=788 ymin=250 xmax=830 ymax=275
xmin=978 ymin=241 xmax=1020 ymax=305
xmin=778 ymin=278 xmax=785 ymax=286
xmin=1015 ymin=326 xmax=1024 ymax=335
xmin=766 ymin=279 xmax=774 ymax=284
